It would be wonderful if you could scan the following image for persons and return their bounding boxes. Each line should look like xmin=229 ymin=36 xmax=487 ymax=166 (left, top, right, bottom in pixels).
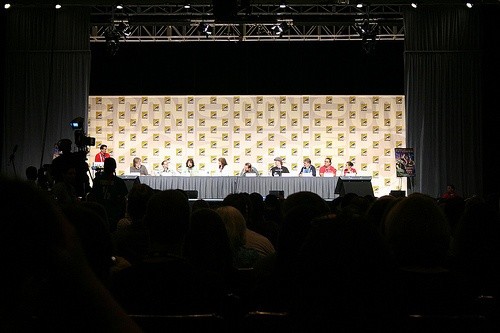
xmin=95 ymin=145 xmax=110 ymax=162
xmin=185 ymin=158 xmax=198 ymax=175
xmin=239 ymin=162 xmax=259 ymax=175
xmin=159 ymin=159 xmax=172 ymax=173
xmin=319 ymin=157 xmax=337 ymax=176
xmin=298 ymin=158 xmax=316 ymax=176
xmin=130 ymin=157 xmax=148 ymax=175
xmin=343 ymin=161 xmax=356 ymax=176
xmin=270 ymin=156 xmax=290 ymax=176
xmin=0 ymin=139 xmax=500 ymax=333
xmin=216 ymin=157 xmax=229 ymax=176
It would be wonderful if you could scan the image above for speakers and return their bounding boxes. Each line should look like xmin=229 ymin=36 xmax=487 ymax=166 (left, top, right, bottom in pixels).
xmin=184 ymin=190 xmax=198 ymax=199
xmin=269 ymin=190 xmax=284 ymax=200
xmin=389 ymin=191 xmax=405 ymax=198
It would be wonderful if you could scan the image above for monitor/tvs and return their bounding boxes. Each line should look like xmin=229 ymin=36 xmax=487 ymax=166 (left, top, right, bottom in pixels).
xmin=334 ymin=176 xmax=372 ymax=194
